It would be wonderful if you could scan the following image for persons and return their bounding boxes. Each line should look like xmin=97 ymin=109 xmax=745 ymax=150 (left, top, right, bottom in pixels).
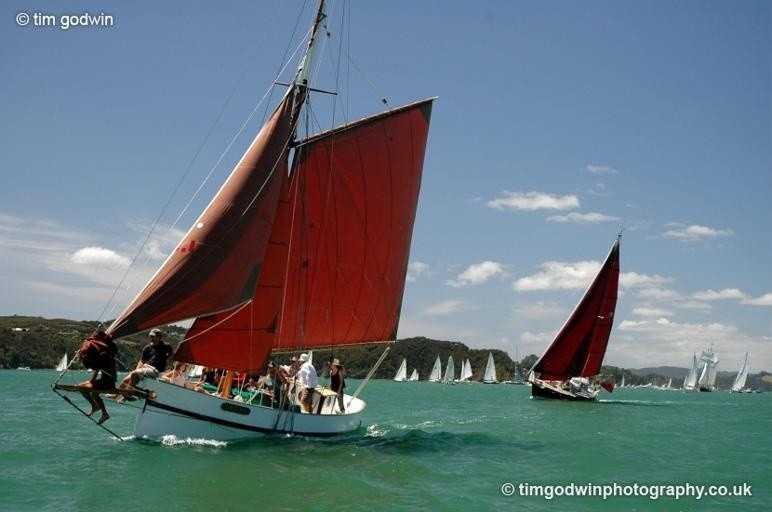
xmin=106 ymin=329 xmax=174 ymax=404
xmin=196 ymin=353 xmax=318 ymax=413
xmin=77 ymin=330 xmax=117 ymax=424
xmin=162 ymin=361 xmax=210 ymax=395
xmin=326 ymin=358 xmax=346 ymax=413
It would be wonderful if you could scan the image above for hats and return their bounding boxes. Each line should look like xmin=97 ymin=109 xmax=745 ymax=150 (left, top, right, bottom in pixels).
xmin=148 ymin=328 xmax=162 ymax=337
xmin=327 ymin=358 xmax=344 ymax=366
xmin=290 ymin=353 xmax=309 ymax=363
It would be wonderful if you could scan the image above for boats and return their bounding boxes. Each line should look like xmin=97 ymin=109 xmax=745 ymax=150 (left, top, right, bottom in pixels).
xmin=17 ymin=364 xmax=34 ymax=373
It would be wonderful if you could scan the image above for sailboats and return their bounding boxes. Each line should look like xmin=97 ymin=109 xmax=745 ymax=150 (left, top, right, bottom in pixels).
xmin=53 ymin=353 xmax=69 ymax=373
xmin=51 ymin=2 xmax=438 ymax=457
xmin=393 ymin=231 xmax=757 ymax=405
